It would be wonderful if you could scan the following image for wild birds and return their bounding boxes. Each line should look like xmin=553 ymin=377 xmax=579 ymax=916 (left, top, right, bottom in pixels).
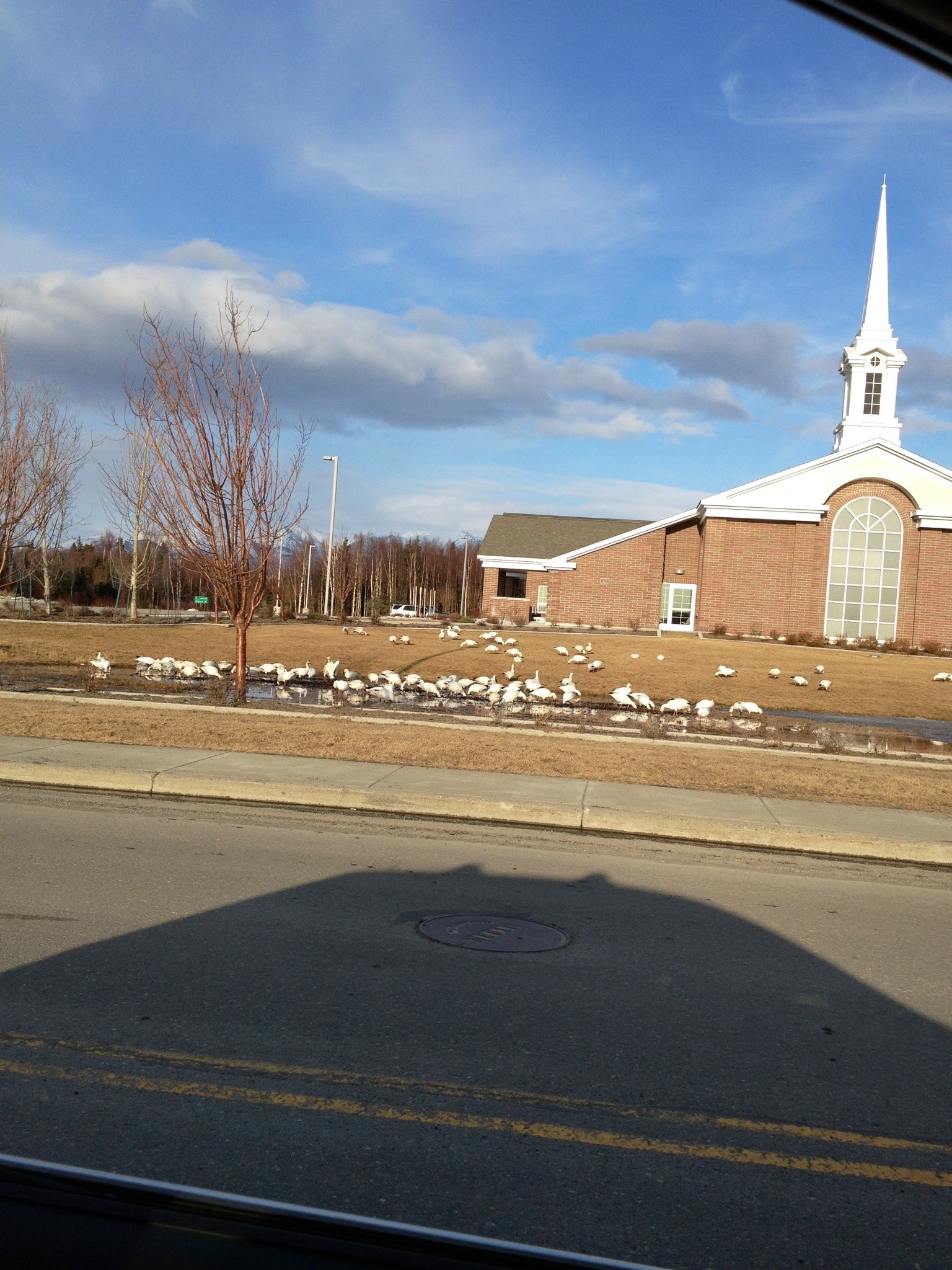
xmin=93 ymin=624 xmax=952 ymax=730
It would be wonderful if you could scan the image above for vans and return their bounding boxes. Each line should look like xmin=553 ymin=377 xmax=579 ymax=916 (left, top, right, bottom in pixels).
xmin=390 ymin=604 xmax=429 ymax=617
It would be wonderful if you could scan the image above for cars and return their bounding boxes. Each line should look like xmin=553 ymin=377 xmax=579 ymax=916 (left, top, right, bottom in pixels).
xmin=424 ymin=605 xmax=450 ymax=618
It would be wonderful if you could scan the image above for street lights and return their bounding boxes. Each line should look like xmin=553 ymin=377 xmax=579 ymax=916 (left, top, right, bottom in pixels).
xmin=460 ymin=536 xmax=469 ymax=616
xmin=322 ymin=455 xmax=338 ymax=615
xmin=302 ymin=545 xmax=317 ymax=616
xmin=273 ymin=532 xmax=283 ymax=617
